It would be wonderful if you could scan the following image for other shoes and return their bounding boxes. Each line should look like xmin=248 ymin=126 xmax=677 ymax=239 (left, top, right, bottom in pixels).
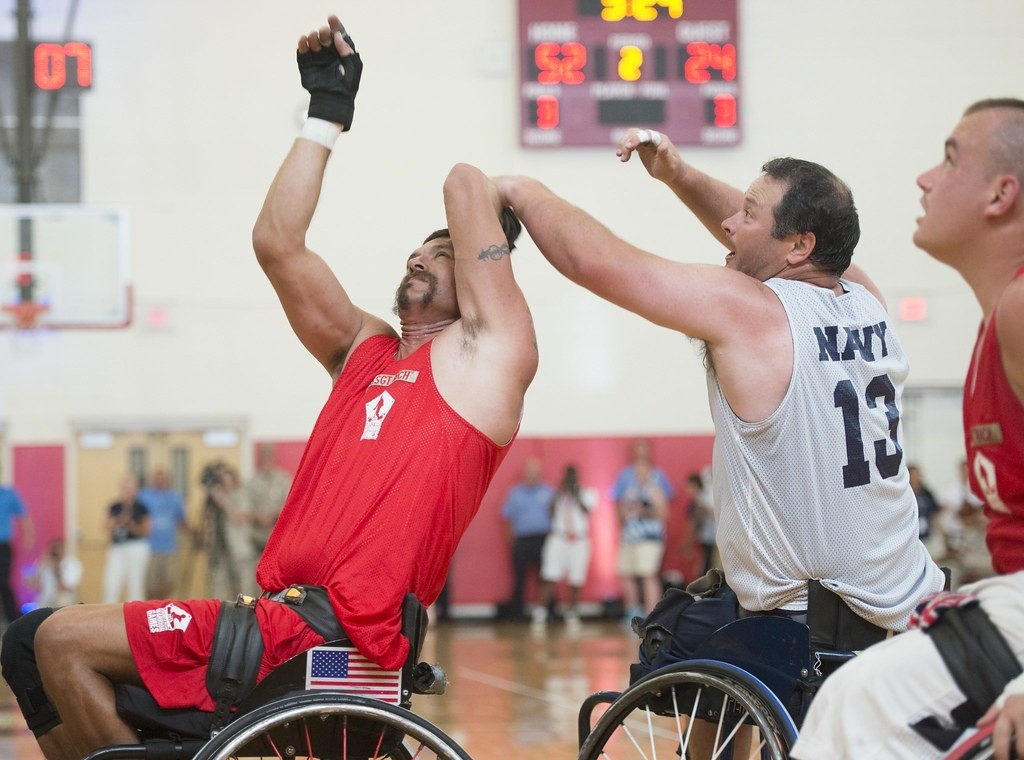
xmin=562 ymin=609 xmax=583 ymax=632
xmin=530 ymin=605 xmax=549 ymax=632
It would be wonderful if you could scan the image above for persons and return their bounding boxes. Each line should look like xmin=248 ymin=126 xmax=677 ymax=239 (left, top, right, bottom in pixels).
xmin=791 ymin=99 xmax=1024 ymax=760
xmin=0 ymin=438 xmax=991 ymax=623
xmin=483 ymin=127 xmax=946 ymax=721
xmin=35 ymin=13 xmax=541 ymax=760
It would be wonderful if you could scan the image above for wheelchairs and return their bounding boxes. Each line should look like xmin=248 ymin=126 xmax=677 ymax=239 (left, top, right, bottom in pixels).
xmin=578 ymin=567 xmax=1024 ymax=760
xmin=80 ymin=594 xmax=474 ymax=760
